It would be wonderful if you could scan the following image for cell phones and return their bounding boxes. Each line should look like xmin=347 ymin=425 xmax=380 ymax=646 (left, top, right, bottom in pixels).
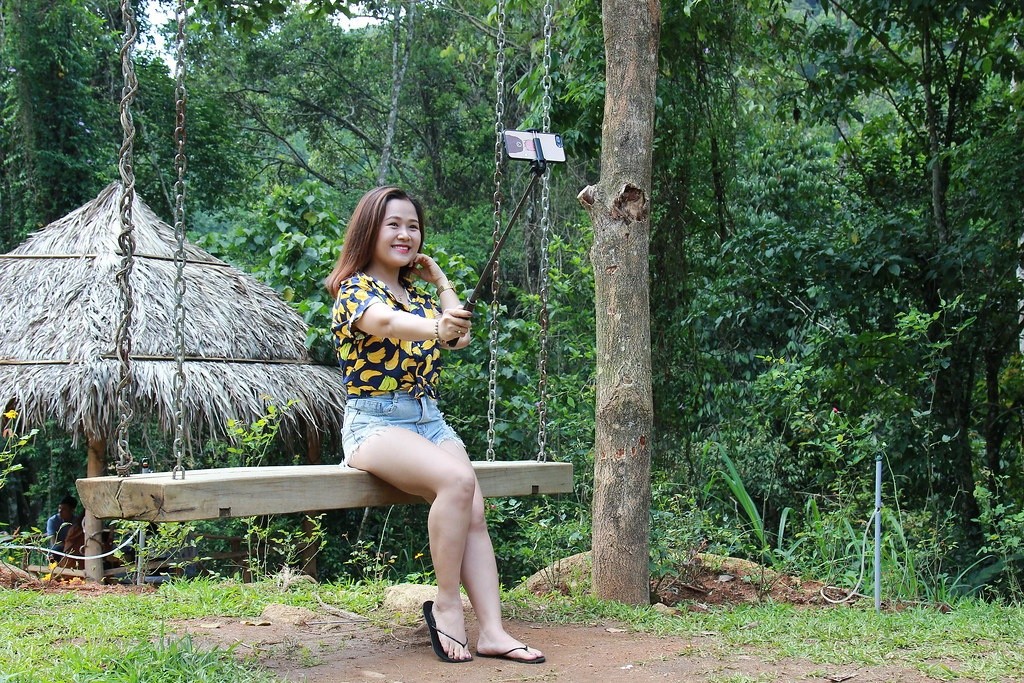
xmin=503 ymin=128 xmax=567 ymax=164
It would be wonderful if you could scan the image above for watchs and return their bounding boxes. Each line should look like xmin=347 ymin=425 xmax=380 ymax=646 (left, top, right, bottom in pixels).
xmin=436 ymin=280 xmax=456 ymax=296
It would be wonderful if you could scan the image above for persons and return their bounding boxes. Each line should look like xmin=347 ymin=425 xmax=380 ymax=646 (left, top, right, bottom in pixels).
xmin=47 ymin=496 xmax=77 ymax=564
xmin=324 ymin=185 xmax=546 ymax=664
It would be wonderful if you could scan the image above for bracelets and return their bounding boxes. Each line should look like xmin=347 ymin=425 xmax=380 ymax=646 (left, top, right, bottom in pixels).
xmin=435 ymin=319 xmax=444 ymax=345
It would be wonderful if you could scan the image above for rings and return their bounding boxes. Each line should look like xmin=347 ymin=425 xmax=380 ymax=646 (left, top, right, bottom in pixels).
xmin=458 ymin=327 xmax=461 ymax=333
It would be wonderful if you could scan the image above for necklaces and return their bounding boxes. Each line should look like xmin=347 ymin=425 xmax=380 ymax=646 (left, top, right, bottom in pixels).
xmin=390 ymin=288 xmax=404 ymax=301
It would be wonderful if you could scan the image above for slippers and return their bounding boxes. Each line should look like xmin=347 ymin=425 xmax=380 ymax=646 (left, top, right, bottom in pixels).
xmin=476 ymin=645 xmax=545 ymax=664
xmin=423 ymin=601 xmax=473 ymax=663
